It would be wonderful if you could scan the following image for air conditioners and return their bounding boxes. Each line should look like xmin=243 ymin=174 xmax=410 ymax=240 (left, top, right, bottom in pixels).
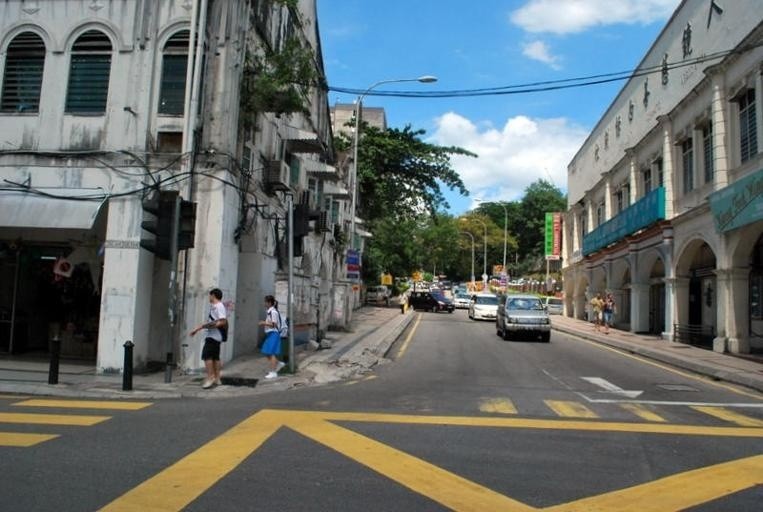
xmin=263 ymin=159 xmax=335 ymax=234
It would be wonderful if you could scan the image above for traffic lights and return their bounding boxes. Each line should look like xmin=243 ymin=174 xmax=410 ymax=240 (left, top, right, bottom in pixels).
xmin=139 ymin=188 xmax=178 ymax=262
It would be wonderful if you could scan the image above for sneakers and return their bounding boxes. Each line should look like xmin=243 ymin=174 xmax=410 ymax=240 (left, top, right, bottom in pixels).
xmin=213 ymin=378 xmax=222 ymax=385
xmin=275 ymin=362 xmax=285 ymax=372
xmin=265 ymin=371 xmax=278 ymax=379
xmin=203 ymin=376 xmax=216 ymax=388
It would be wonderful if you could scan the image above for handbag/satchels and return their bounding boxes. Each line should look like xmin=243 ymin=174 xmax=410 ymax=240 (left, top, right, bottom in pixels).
xmin=218 ymin=319 xmax=228 ymax=342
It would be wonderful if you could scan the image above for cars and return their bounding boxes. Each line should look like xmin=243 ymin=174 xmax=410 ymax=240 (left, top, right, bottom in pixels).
xmin=364 ymin=274 xmax=472 ymax=316
xmin=467 ymin=293 xmax=502 ymax=320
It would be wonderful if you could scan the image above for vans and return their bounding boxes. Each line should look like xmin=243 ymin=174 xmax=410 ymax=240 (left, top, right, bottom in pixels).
xmin=540 ymin=295 xmax=566 ymax=317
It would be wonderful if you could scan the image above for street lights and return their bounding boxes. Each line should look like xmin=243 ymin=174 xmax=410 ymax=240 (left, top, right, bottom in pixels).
xmin=451 ymin=196 xmax=509 ymax=292
xmin=346 ymin=75 xmax=441 ymax=282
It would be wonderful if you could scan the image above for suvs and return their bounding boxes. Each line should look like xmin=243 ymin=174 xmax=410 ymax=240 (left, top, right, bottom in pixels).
xmin=495 ymin=294 xmax=554 ymax=342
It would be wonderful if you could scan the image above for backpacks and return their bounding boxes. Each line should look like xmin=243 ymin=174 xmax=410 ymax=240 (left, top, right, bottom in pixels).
xmin=267 ymin=308 xmax=288 ymax=339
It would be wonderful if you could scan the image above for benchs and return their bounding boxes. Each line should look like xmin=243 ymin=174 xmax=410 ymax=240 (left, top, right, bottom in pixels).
xmin=674 ymin=322 xmax=713 ymax=348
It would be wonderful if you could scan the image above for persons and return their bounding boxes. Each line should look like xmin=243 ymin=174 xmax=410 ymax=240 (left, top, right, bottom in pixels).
xmin=602 ymin=292 xmax=617 ymax=334
xmin=396 ymin=291 xmax=407 ymax=314
xmin=256 ymin=294 xmax=286 ymax=379
xmin=589 ymin=292 xmax=605 ymax=331
xmin=186 ymin=288 xmax=229 ymax=390
